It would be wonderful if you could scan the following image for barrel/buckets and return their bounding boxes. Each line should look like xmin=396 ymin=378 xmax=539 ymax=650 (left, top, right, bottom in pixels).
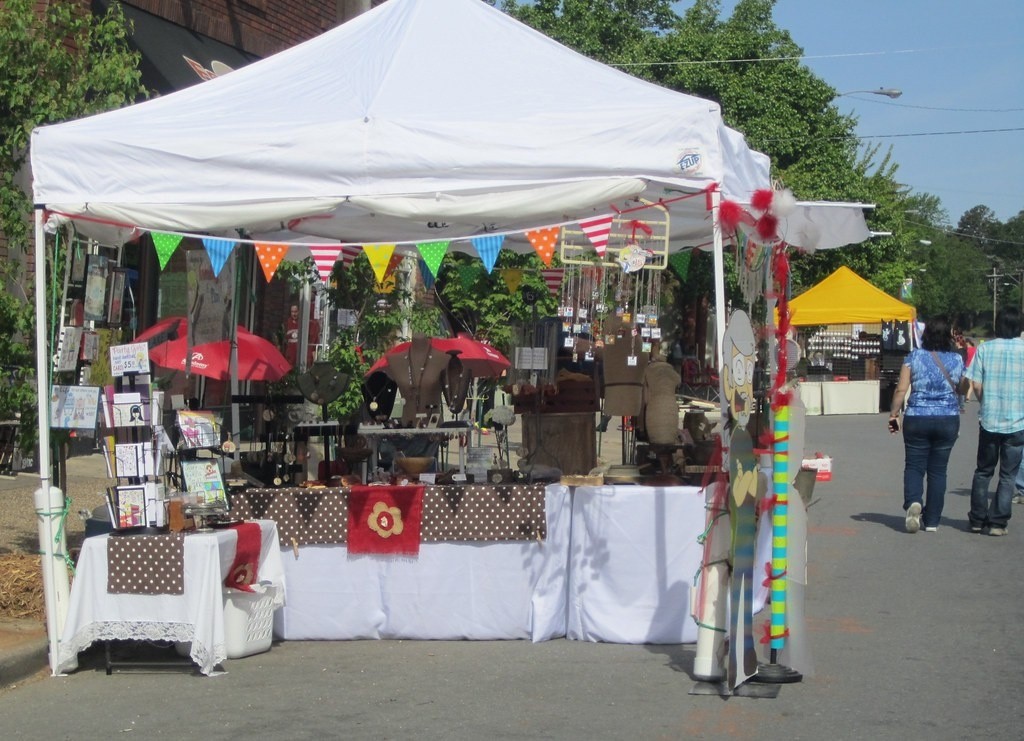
xmin=305 ymin=434 xmax=335 ymax=486
xmin=557 ymin=379 xmax=600 ymax=411
xmin=792 ymin=466 xmax=817 ymax=512
xmin=520 ymin=410 xmax=598 ymax=483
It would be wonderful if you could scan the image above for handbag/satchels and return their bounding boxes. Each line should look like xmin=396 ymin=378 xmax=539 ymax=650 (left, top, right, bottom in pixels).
xmin=957 ymin=375 xmax=970 ymax=395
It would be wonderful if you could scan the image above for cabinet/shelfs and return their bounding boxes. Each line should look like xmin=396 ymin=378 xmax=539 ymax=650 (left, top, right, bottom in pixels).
xmin=171 ymin=421 xmax=232 ymax=528
xmin=99 ymin=369 xmax=173 ymax=533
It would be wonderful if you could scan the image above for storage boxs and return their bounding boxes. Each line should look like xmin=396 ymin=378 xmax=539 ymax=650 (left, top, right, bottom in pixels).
xmin=802 ymin=457 xmax=832 ymax=481
xmin=175 ymin=587 xmax=277 ymax=659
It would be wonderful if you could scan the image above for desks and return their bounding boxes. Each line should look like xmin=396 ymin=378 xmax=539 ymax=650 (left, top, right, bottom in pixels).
xmin=53 ymin=519 xmax=287 ymax=677
xmin=798 ymin=382 xmax=822 ymax=415
xmin=566 ymin=485 xmax=774 ymax=643
xmin=822 ymin=380 xmax=880 ymax=414
xmin=212 ymin=486 xmax=571 ymax=644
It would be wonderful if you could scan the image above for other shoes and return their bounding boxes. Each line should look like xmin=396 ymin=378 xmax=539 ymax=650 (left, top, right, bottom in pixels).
xmin=971 ymin=526 xmax=983 ymax=531
xmin=988 ymin=528 xmax=1008 ymax=535
xmin=926 ymin=525 xmax=939 ymax=532
xmin=905 ymin=502 xmax=922 ymax=533
xmin=1012 ymin=496 xmax=1024 ymax=504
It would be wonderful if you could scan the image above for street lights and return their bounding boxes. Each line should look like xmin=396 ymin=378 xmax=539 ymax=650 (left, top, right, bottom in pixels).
xmin=840 ymin=85 xmax=903 ymax=99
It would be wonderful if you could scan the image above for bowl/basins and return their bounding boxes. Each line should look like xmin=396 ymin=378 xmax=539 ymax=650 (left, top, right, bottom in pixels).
xmin=393 ymin=455 xmax=436 ymax=477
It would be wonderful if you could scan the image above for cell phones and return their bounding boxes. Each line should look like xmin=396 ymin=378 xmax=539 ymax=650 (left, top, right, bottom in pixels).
xmin=889 ymin=419 xmax=899 ymax=433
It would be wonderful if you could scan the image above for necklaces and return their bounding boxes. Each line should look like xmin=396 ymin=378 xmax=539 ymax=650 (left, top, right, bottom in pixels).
xmin=310 ymin=370 xmax=339 ymax=404
xmin=506 ymin=320 xmax=562 ymax=397
xmin=556 ymin=218 xmax=663 ymax=368
xmin=445 ymin=367 xmax=462 ymax=418
xmin=364 ymin=380 xmax=390 ymax=412
xmin=247 ymin=377 xmax=305 ymax=487
xmin=406 ymin=342 xmax=432 ymax=412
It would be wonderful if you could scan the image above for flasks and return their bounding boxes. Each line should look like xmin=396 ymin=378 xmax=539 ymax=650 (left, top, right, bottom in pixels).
xmin=357 ymin=456 xmax=368 ymax=487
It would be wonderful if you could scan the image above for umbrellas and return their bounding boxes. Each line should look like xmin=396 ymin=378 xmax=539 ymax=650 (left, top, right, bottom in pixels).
xmin=125 ymin=317 xmax=292 ymax=409
xmin=363 ymin=336 xmax=512 ymax=379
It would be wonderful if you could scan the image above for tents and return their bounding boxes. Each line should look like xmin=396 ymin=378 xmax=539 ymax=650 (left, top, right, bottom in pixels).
xmin=769 ymin=265 xmax=916 ymax=325
xmin=30 ymin=0 xmax=773 ymax=686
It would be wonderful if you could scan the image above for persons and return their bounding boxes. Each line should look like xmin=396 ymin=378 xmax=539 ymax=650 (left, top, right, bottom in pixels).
xmin=284 ymin=304 xmax=321 ymax=369
xmin=954 ymin=306 xmax=1023 ymax=534
xmin=385 ymin=332 xmax=451 ymax=428
xmin=598 ymin=306 xmax=652 ymax=418
xmin=1011 ymin=459 xmax=1024 ymax=505
xmin=888 ymin=314 xmax=966 ymax=533
xmin=949 ymin=327 xmax=977 ymax=411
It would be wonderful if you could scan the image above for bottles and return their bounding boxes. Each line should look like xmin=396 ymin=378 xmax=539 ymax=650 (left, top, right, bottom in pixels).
xmin=281 ymin=433 xmax=295 ymax=489
xmin=259 ymin=429 xmax=283 ymax=489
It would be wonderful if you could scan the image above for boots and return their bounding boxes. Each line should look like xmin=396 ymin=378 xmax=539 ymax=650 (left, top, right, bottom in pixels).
xmin=596 ymin=415 xmax=611 ymax=432
xmin=617 ymin=425 xmax=622 ymax=430
